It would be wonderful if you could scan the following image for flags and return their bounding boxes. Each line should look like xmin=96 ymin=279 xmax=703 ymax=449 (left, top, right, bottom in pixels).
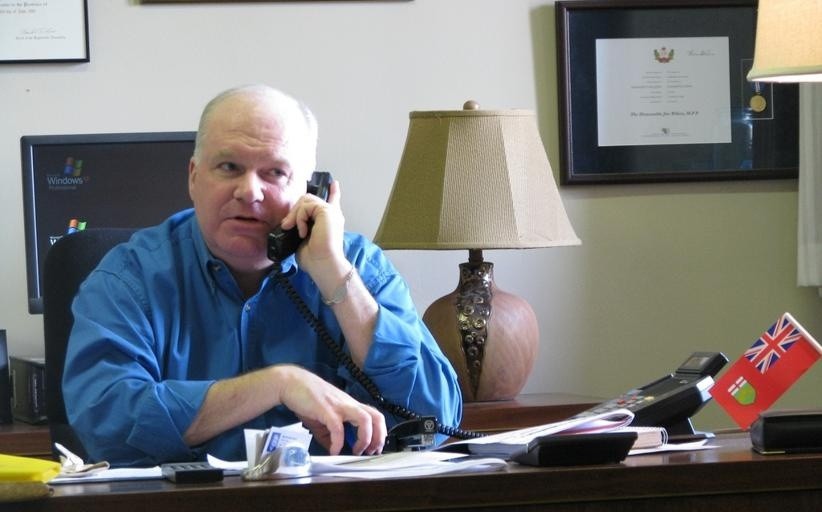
xmin=708 ymin=315 xmax=821 ymax=433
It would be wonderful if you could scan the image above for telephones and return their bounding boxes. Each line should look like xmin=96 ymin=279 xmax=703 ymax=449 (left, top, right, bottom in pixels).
xmin=267 ymin=173 xmax=341 ymax=263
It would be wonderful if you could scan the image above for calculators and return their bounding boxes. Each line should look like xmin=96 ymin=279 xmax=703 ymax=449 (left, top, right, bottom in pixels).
xmin=511 ymin=431 xmax=638 ymax=465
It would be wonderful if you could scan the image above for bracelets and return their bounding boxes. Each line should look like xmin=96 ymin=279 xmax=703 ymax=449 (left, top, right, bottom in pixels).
xmin=319 ymin=265 xmax=355 ymax=308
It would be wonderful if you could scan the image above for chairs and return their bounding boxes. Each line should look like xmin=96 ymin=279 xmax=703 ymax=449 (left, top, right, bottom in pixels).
xmin=34 ymin=229 xmax=152 ymax=455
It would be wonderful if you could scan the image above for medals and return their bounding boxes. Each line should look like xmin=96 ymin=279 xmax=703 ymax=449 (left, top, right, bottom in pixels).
xmin=749 ymin=94 xmax=767 ymax=112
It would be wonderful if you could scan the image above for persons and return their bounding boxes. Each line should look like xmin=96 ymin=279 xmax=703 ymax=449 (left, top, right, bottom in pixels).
xmin=59 ymin=84 xmax=464 ymax=467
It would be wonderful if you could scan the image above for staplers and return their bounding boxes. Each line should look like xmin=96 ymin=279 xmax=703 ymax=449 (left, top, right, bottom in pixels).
xmin=387 ymin=416 xmax=438 ymax=453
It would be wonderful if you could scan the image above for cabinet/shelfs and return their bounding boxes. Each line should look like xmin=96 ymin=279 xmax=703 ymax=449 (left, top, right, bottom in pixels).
xmin=453 ymin=392 xmax=603 ymax=431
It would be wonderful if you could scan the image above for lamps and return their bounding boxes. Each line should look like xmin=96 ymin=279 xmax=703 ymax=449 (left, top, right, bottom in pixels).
xmin=746 ymin=0 xmax=818 ymax=84
xmin=371 ymin=101 xmax=584 ymax=401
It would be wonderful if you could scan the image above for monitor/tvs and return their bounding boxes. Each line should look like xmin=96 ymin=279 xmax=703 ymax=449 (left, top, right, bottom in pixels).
xmin=20 ymin=132 xmax=197 ymax=314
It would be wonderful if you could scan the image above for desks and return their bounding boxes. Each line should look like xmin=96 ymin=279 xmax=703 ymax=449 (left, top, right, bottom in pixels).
xmin=1 ymin=425 xmax=822 ymax=511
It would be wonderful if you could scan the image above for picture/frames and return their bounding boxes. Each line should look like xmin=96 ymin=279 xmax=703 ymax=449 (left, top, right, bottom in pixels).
xmin=554 ymin=1 xmax=799 ymax=186
xmin=0 ymin=0 xmax=92 ymax=65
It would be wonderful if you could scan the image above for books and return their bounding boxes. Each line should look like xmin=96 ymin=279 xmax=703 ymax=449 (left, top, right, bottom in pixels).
xmin=468 ymin=428 xmax=667 ymax=455
